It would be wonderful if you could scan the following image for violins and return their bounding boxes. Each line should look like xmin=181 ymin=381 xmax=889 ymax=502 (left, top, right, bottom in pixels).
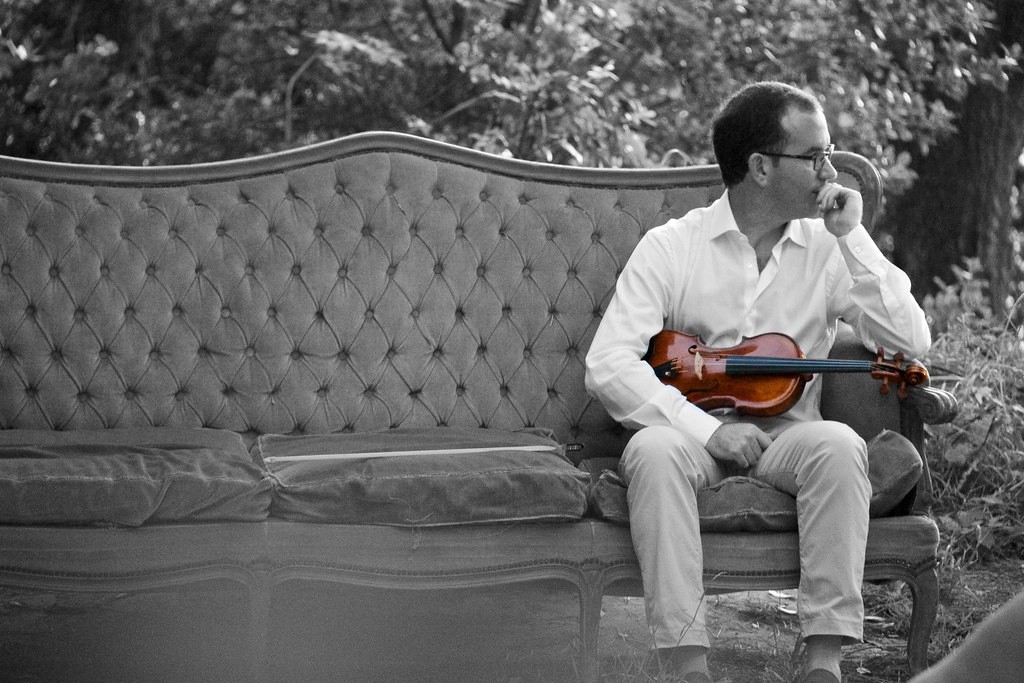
xmin=644 ymin=327 xmax=931 ymax=419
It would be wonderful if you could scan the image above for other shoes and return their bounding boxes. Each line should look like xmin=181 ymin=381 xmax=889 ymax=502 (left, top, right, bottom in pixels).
xmin=798 ymin=669 xmax=839 ymax=683
xmin=685 ymin=671 xmax=713 ymax=683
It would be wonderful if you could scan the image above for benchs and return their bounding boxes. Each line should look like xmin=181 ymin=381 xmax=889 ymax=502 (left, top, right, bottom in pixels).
xmin=0 ymin=130 xmax=960 ymax=683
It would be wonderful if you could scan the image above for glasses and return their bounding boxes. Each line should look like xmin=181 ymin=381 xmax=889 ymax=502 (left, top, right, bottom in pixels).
xmin=760 ymin=144 xmax=835 ymax=170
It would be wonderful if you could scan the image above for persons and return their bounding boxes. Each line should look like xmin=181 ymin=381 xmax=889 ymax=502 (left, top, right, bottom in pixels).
xmin=585 ymin=81 xmax=933 ymax=682
